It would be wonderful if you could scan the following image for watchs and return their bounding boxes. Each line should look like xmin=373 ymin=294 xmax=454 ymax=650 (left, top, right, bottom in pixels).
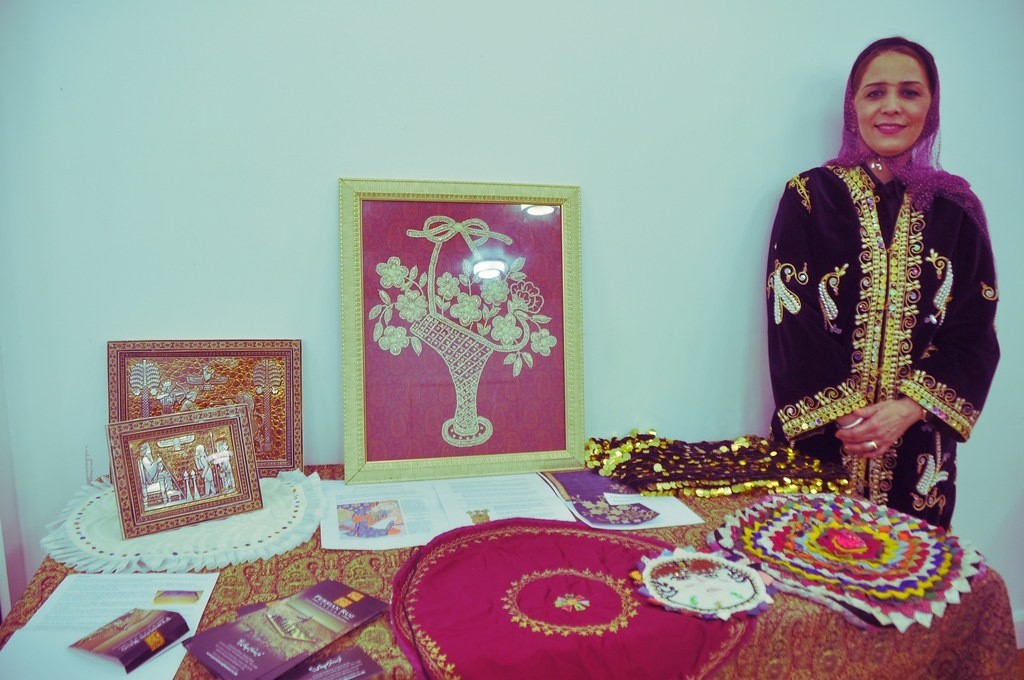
xmin=921 ymin=408 xmax=933 ymax=423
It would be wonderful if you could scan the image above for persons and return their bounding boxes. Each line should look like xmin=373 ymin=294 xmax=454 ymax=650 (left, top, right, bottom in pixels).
xmin=766 ymin=36 xmax=1001 ymax=533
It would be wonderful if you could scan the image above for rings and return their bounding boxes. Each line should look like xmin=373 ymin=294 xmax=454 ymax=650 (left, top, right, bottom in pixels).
xmin=870 ymin=441 xmax=878 ymax=451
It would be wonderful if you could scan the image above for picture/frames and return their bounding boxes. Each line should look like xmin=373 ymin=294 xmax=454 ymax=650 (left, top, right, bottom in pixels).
xmin=106 ymin=338 xmax=304 ymax=479
xmin=338 ymin=177 xmax=585 ymax=486
xmin=106 ymin=403 xmax=265 ymax=540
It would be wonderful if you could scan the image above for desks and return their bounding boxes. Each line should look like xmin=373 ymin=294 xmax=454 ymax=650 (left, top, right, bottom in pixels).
xmin=0 ymin=428 xmax=1018 ymax=680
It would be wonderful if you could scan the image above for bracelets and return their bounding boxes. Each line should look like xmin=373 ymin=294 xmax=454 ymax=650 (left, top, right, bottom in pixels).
xmin=836 ymin=416 xmax=863 ymax=429
